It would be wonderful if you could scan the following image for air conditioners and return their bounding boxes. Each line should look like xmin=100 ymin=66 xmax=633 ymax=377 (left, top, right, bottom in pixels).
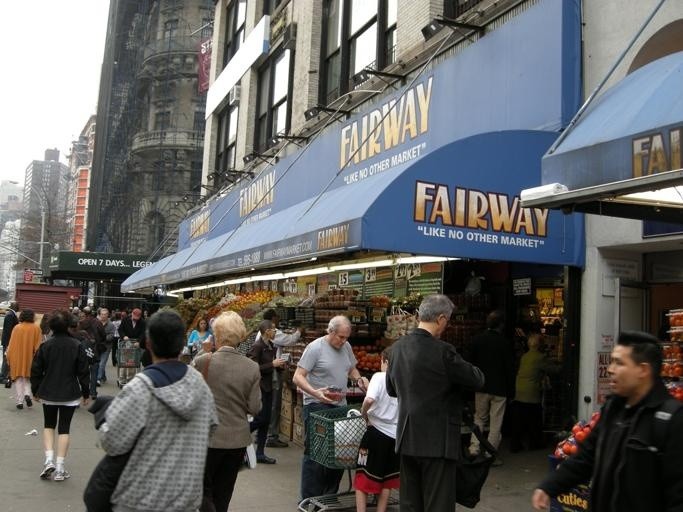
xmin=229 ymin=87 xmax=240 ymax=107
xmin=282 ymin=22 xmax=296 ymax=51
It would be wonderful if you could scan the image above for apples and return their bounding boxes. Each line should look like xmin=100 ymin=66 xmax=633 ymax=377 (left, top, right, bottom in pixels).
xmin=371 ymin=295 xmax=390 ymax=307
xmin=352 ymin=345 xmax=379 ymax=352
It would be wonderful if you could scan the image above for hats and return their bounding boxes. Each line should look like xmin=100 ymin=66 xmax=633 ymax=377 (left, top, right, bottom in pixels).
xmin=132 ymin=308 xmax=142 ymax=320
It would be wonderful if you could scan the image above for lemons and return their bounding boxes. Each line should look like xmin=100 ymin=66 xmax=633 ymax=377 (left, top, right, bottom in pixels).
xmin=229 ymin=289 xmax=279 ymax=310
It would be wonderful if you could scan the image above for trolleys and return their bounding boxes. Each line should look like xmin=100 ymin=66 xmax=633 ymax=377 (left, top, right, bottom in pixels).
xmin=116 ymin=338 xmax=142 ymax=387
xmin=295 ymin=403 xmax=402 ymax=511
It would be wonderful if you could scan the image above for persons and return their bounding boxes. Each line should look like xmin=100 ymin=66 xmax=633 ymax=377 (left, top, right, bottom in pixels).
xmin=1 ymin=300 xmax=152 ymax=481
xmin=188 ymin=308 xmax=305 ymax=464
xmin=98 ymin=310 xmax=219 ymax=512
xmin=527 ymin=328 xmax=682 ymax=512
xmin=191 ymin=311 xmax=262 ymax=511
xmin=293 ymin=293 xmax=572 ymax=512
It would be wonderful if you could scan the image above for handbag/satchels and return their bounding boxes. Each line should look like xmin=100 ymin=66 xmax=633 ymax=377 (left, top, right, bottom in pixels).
xmin=457 ymin=441 xmax=496 ymax=509
xmin=384 ymin=312 xmax=418 ymax=339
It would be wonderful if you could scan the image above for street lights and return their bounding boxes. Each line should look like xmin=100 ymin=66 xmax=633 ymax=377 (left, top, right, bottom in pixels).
xmin=8 ymin=179 xmax=49 ymax=270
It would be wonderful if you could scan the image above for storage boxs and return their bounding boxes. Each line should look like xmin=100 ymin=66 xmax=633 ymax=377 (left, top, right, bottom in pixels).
xmin=280 ymin=385 xmax=307 ymax=448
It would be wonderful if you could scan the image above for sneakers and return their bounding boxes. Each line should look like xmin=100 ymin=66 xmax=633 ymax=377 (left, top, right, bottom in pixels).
xmin=266 ymin=441 xmax=287 ymax=447
xmin=24 ymin=395 xmax=32 ymax=407
xmin=40 ymin=463 xmax=56 ymax=478
xmin=259 ymin=456 xmax=277 ymax=464
xmin=54 ymin=470 xmax=70 ymax=481
